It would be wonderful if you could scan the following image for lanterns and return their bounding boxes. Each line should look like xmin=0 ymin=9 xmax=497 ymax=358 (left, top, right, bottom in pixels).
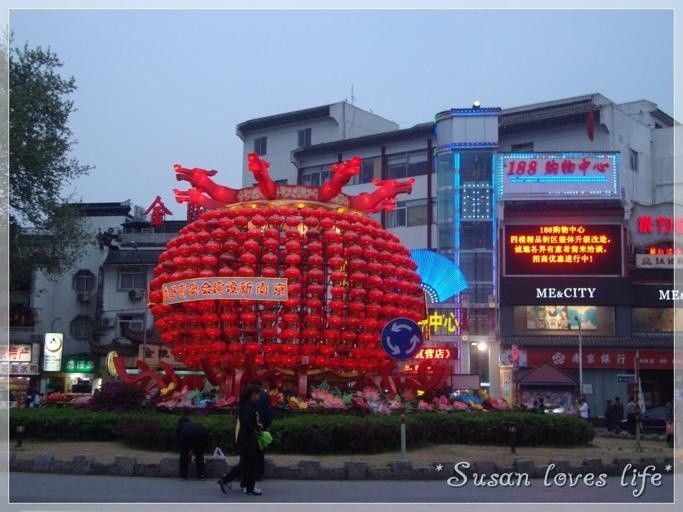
xmin=147 ymin=207 xmax=427 ymax=375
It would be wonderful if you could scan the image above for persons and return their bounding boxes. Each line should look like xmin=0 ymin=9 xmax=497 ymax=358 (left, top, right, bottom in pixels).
xmin=535 ymin=304 xmax=571 ymax=331
xmin=575 ymin=397 xmax=591 ymax=423
xmin=605 ymin=397 xmax=639 ymax=436
xmin=217 ymin=386 xmax=263 ymax=495
xmin=666 ymin=399 xmax=674 ymax=448
xmin=9 ymin=388 xmax=44 ymax=408
xmin=249 ymin=378 xmax=273 ymax=492
xmin=178 ymin=425 xmax=209 ymax=481
xmin=532 ymin=396 xmax=546 ymax=412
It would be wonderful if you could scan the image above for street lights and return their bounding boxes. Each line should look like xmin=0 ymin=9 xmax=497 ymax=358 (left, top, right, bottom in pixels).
xmin=573 ymin=315 xmax=582 ymax=399
xmin=129 ymin=240 xmax=147 ymax=362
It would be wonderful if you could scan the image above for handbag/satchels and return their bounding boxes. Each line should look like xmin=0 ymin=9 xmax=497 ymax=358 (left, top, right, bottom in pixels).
xmin=206 ymin=457 xmax=228 ymax=478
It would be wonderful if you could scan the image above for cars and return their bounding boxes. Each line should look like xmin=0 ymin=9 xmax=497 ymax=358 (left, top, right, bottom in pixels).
xmin=621 ymin=406 xmax=666 ymax=433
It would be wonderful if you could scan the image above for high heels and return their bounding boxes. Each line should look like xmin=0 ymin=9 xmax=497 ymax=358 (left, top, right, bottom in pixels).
xmin=247 ymin=488 xmax=261 ymax=495
xmin=215 ymin=479 xmax=227 ymax=495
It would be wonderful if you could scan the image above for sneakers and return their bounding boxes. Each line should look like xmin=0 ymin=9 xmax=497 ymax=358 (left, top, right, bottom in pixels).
xmin=200 ymin=474 xmax=208 ymax=480
xmin=175 ymin=474 xmax=185 ymax=481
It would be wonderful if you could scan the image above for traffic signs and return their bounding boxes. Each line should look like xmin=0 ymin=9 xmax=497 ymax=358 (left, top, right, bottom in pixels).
xmin=617 ymin=374 xmax=637 ymax=383
xmin=380 ymin=317 xmax=423 ymax=360
